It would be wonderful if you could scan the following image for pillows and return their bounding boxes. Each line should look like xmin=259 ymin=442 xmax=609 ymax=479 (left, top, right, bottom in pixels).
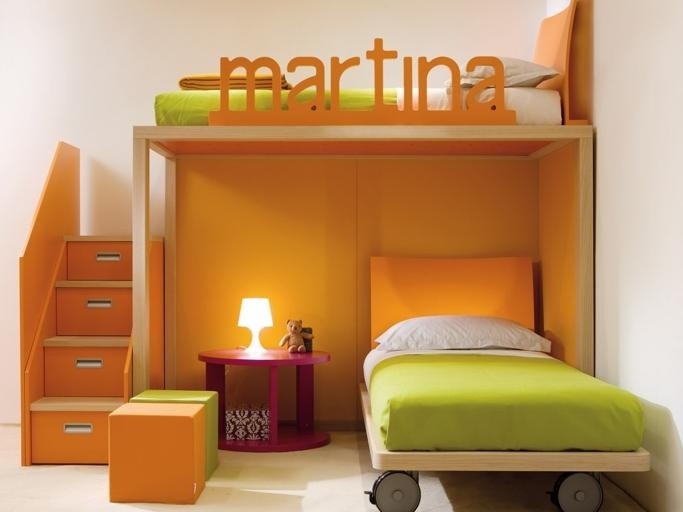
xmin=446 ymin=59 xmax=559 ymax=90
xmin=374 ymin=317 xmax=553 ymax=355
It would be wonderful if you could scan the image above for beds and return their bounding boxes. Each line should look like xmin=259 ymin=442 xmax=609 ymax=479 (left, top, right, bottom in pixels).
xmin=360 ymin=255 xmax=653 ymax=510
xmin=133 ymin=0 xmax=596 ymax=395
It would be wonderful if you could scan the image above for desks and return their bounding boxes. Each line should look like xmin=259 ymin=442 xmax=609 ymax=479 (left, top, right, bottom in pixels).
xmin=197 ymin=351 xmax=334 ymax=451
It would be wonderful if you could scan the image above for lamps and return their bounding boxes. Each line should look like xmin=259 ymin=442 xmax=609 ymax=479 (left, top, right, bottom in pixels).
xmin=233 ymin=297 xmax=274 ymax=358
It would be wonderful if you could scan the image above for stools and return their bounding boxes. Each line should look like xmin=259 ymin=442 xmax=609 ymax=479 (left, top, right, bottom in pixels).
xmin=130 ymin=389 xmax=218 ymax=476
xmin=108 ymin=400 xmax=206 ymax=506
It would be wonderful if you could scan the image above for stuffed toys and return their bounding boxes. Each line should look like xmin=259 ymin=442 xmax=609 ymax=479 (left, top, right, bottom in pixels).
xmin=279 ymin=319 xmax=314 ymax=353
